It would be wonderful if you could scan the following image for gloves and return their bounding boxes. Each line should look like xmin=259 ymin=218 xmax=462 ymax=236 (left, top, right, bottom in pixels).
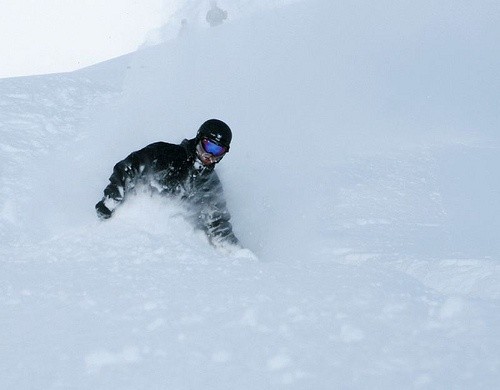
xmin=95 ymin=200 xmax=112 ymax=219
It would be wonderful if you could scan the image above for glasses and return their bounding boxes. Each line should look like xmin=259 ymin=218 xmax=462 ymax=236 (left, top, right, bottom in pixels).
xmin=200 ymin=131 xmax=228 ymax=157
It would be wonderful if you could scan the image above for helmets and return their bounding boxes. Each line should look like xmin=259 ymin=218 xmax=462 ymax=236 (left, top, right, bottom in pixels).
xmin=199 ymin=118 xmax=232 ymax=152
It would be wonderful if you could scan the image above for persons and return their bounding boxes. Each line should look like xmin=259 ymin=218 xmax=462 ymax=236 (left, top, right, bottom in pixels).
xmin=95 ymin=118 xmax=245 ymax=256
